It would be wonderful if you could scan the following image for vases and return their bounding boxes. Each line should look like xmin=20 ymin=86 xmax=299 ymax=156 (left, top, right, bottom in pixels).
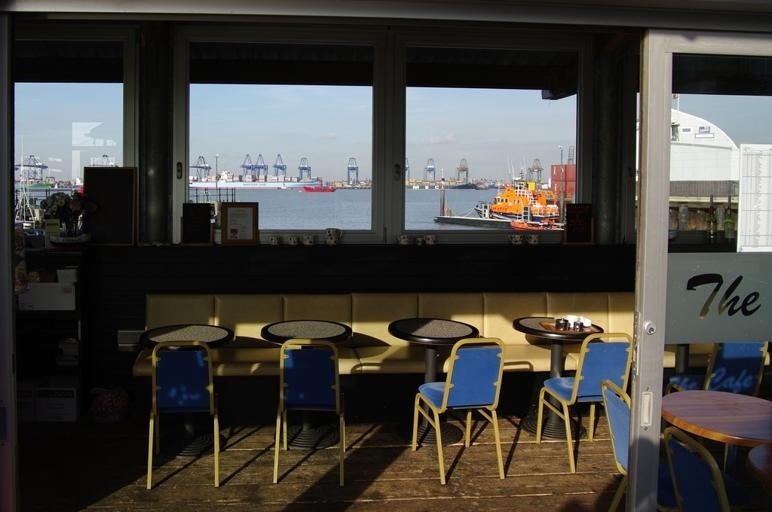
xmin=60 ymin=216 xmax=82 ymax=238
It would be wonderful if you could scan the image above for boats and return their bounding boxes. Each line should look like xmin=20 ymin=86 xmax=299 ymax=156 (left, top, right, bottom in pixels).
xmin=474 ymin=156 xmax=560 ymax=224
xmin=188 ymin=169 xmax=329 ymax=191
xmin=303 ymin=176 xmax=336 ymax=193
xmin=510 ymin=218 xmax=564 ymax=231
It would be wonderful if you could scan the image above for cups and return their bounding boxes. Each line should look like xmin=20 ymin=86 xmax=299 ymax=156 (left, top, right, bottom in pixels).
xmin=564 ymin=314 xmax=593 ymax=328
xmin=265 ymin=227 xmax=344 ymax=246
xmin=396 ymin=234 xmax=436 ymax=244
xmin=505 ymin=233 xmax=539 ymax=246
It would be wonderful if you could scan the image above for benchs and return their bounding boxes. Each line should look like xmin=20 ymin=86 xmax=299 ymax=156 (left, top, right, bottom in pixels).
xmin=131 ymin=290 xmax=714 ymax=380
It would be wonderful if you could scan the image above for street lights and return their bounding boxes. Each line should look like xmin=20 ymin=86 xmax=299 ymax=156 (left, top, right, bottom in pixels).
xmin=215 ymin=153 xmax=220 ymax=200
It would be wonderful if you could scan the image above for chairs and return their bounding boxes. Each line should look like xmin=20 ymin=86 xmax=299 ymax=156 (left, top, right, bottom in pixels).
xmin=536 ymin=331 xmax=633 ymax=477
xmin=600 ymin=378 xmax=745 ymax=510
xmin=410 ymin=332 xmax=514 ymax=485
xmin=660 ymin=424 xmax=744 ymax=511
xmin=666 ymin=342 xmax=769 ymax=396
xmin=271 ymin=339 xmax=347 ymax=485
xmin=145 ymin=341 xmax=221 ymax=490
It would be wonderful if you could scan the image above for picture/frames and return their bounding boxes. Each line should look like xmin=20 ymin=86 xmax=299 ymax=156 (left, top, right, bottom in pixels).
xmin=222 ymin=201 xmax=259 ymax=244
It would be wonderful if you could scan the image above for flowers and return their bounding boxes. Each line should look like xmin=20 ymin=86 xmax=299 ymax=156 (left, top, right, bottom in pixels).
xmin=39 ymin=187 xmax=86 ymax=222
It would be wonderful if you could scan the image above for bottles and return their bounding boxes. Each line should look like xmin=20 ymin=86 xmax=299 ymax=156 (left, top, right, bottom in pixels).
xmin=555 ymin=316 xmax=568 ymax=332
xmin=668 ymin=193 xmax=736 ymax=244
xmin=573 ymin=320 xmax=584 ymax=332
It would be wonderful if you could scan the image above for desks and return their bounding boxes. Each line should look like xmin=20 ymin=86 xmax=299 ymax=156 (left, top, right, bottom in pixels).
xmin=659 ymin=388 xmax=771 ymax=448
xmin=261 ymin=318 xmax=352 ymax=449
xmin=387 ymin=315 xmax=480 ymax=444
xmin=746 ymin=441 xmax=772 ymax=496
xmin=513 ymin=315 xmax=605 ymax=440
xmin=141 ymin=324 xmax=234 ymax=460
